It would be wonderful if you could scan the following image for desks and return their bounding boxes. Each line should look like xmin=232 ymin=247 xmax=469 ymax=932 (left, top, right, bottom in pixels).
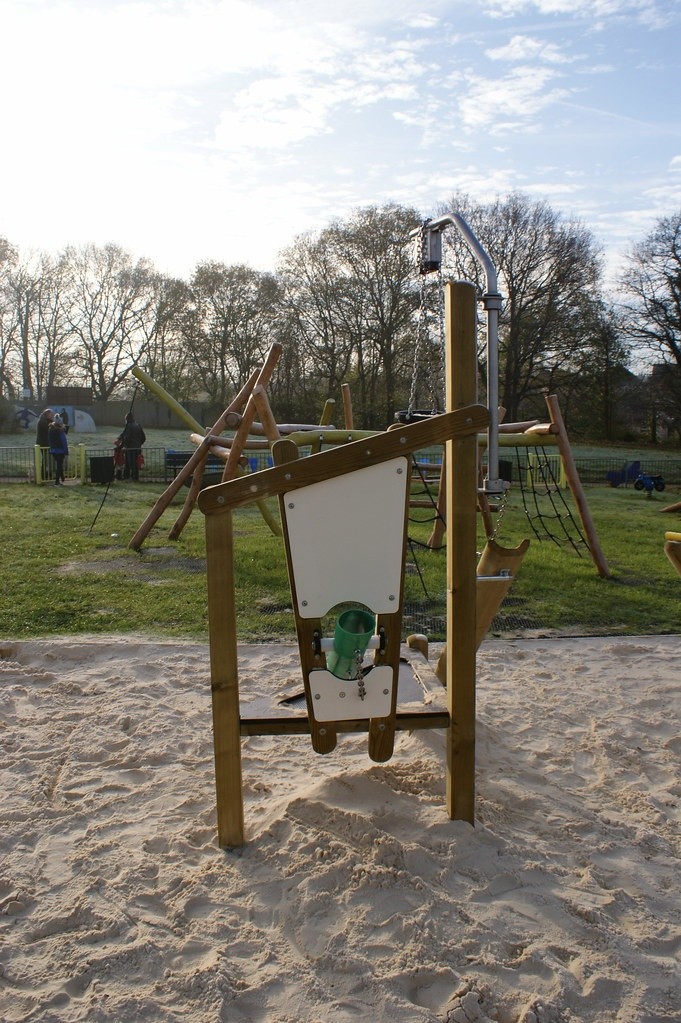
xmin=411 ymin=463 xmax=489 ymax=478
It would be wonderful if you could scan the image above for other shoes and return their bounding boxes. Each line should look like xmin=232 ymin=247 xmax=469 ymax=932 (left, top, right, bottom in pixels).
xmin=55 ymin=481 xmax=63 ymax=485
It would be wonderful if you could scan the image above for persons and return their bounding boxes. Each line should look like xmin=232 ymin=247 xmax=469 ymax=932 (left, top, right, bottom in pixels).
xmin=47 ymin=413 xmax=69 ymax=487
xmin=35 ymin=409 xmax=53 ymax=480
xmin=113 ymin=439 xmax=126 ymax=480
xmin=117 ymin=411 xmax=146 ymax=481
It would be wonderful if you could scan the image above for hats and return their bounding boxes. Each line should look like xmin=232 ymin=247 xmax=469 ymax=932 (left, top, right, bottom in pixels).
xmin=54 ymin=413 xmax=63 ymax=425
xmin=125 ymin=412 xmax=133 ymax=421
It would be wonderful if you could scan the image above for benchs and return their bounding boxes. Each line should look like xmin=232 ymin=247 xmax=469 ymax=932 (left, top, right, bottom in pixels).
xmin=164 ymin=451 xmax=225 ymax=480
xmin=411 ymin=475 xmax=441 ymax=485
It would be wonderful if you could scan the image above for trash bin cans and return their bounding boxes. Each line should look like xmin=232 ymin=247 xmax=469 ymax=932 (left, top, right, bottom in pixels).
xmin=498 ymin=460 xmax=512 ymax=482
xmin=89 ymin=455 xmax=115 ymax=485
